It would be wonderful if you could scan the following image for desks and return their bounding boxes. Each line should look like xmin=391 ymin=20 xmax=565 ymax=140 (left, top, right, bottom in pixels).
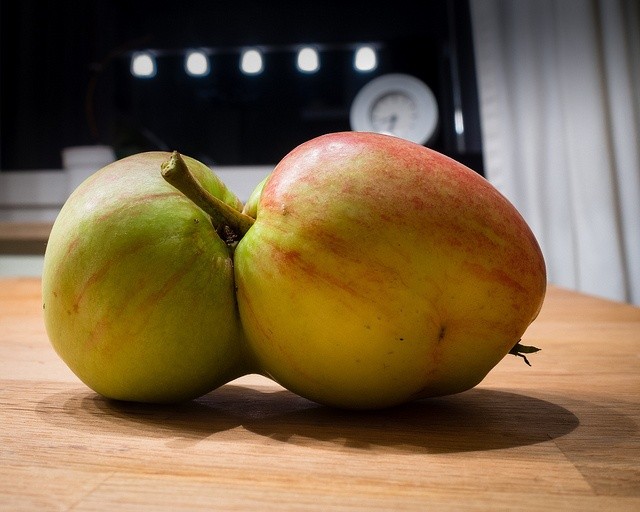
xmin=1 ymin=277 xmax=639 ymax=511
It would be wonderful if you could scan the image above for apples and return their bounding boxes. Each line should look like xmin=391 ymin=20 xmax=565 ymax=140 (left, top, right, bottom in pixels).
xmin=41 ymin=131 xmax=236 ymax=407
xmin=242 ymin=130 xmax=559 ymax=413
